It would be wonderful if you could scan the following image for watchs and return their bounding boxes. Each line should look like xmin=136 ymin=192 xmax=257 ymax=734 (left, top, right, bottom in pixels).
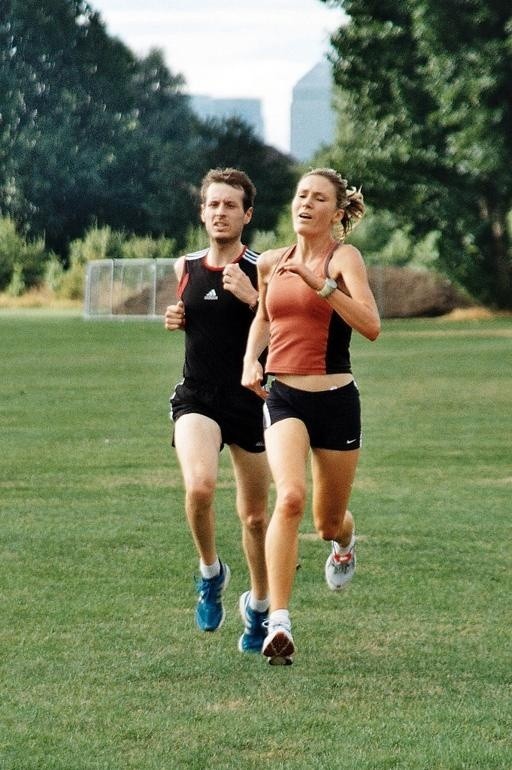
xmin=249 ymin=297 xmax=259 ymax=313
xmin=317 ymin=277 xmax=339 ymax=298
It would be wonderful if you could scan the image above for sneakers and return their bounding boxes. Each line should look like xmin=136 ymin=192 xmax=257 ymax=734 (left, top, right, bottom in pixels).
xmin=195 ymin=562 xmax=230 ymax=633
xmin=260 ymin=629 xmax=296 ymax=666
xmin=324 ymin=511 xmax=356 ymax=591
xmin=237 ymin=591 xmax=269 ymax=657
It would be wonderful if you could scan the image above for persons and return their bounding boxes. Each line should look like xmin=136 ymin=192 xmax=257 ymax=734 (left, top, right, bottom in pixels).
xmin=238 ymin=168 xmax=380 ymax=667
xmin=164 ymin=167 xmax=276 ymax=657
